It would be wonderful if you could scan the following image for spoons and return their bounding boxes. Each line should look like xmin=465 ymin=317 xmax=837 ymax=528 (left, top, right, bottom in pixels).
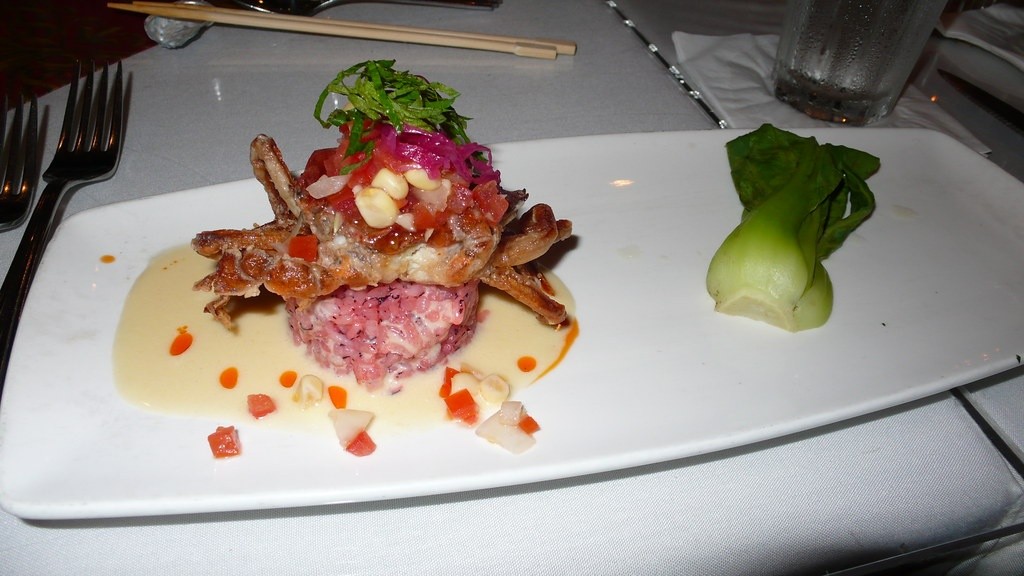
xmin=242 ymin=0 xmax=498 ymax=19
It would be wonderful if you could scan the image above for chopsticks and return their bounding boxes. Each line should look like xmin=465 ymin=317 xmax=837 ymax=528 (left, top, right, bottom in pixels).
xmin=107 ymin=0 xmax=578 ymax=60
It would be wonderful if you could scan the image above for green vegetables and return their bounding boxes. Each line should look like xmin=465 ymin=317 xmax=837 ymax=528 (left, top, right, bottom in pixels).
xmin=706 ymin=121 xmax=882 ymax=334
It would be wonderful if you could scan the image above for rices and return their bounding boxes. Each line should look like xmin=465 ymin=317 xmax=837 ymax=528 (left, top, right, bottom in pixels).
xmin=284 ymin=278 xmax=483 ymax=387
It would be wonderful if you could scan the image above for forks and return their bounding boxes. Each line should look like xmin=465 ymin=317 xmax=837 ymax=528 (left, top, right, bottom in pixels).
xmin=1 ymin=59 xmax=124 ymax=402
xmin=0 ymin=91 xmax=41 ymax=238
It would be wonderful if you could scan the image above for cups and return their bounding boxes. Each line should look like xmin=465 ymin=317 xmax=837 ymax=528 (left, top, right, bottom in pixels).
xmin=769 ymin=0 xmax=951 ymax=126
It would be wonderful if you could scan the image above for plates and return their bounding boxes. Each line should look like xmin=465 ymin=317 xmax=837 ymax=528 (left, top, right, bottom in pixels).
xmin=0 ymin=124 xmax=1024 ymax=523
